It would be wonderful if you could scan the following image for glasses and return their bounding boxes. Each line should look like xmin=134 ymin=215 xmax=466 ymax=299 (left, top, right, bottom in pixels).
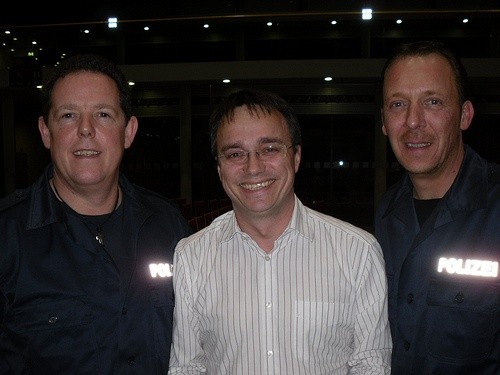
xmin=215 ymin=143 xmax=297 ymax=165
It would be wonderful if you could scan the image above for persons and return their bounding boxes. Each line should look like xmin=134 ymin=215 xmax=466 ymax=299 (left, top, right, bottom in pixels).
xmin=163 ymin=88 xmax=393 ymax=375
xmin=0 ymin=55 xmax=192 ymax=375
xmin=374 ymin=39 xmax=500 ymax=375
